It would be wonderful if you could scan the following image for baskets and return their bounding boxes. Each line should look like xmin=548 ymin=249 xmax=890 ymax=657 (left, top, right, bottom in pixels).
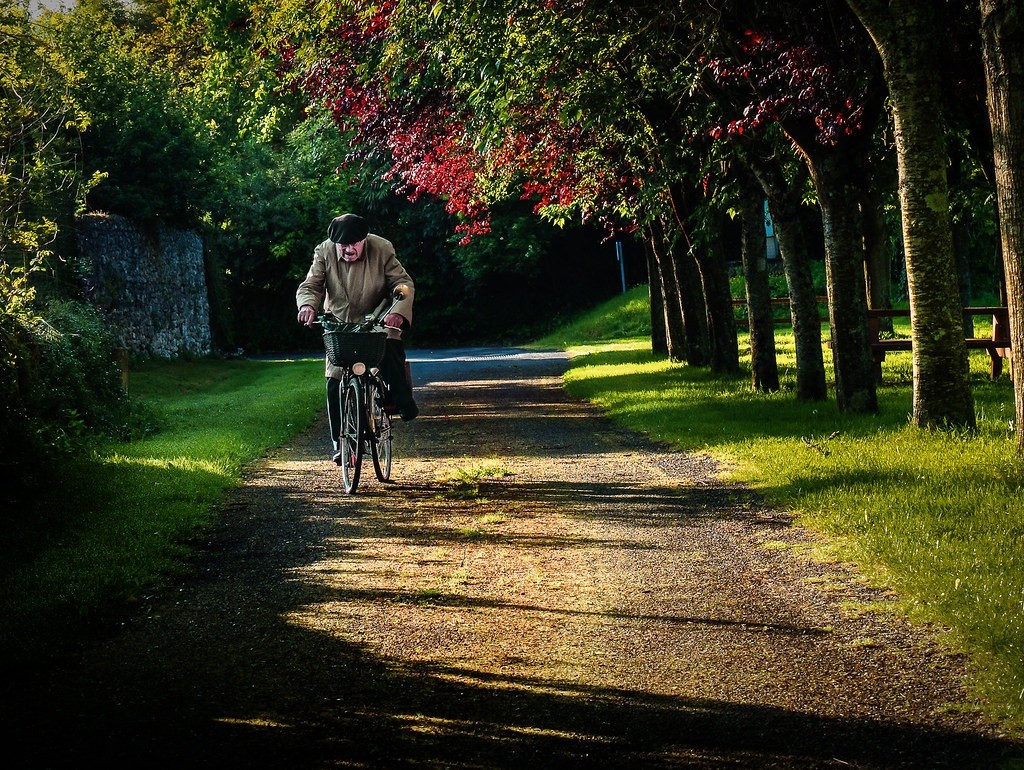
xmin=322 ymin=332 xmax=388 ymax=367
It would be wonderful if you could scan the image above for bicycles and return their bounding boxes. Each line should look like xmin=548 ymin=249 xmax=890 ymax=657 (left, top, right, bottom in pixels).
xmin=303 ymin=277 xmax=409 ymax=495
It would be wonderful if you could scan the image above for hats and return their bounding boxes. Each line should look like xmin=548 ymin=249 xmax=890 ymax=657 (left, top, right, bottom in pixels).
xmin=328 ymin=214 xmax=370 ymax=244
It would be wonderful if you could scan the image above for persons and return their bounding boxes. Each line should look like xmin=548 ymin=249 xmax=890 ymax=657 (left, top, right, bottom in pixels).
xmin=295 ymin=214 xmax=419 ymax=468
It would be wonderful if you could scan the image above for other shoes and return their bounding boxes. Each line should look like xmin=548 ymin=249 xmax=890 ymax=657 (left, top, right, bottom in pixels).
xmin=399 ymin=398 xmax=419 ymax=422
xmin=333 ymin=449 xmax=360 ymax=464
